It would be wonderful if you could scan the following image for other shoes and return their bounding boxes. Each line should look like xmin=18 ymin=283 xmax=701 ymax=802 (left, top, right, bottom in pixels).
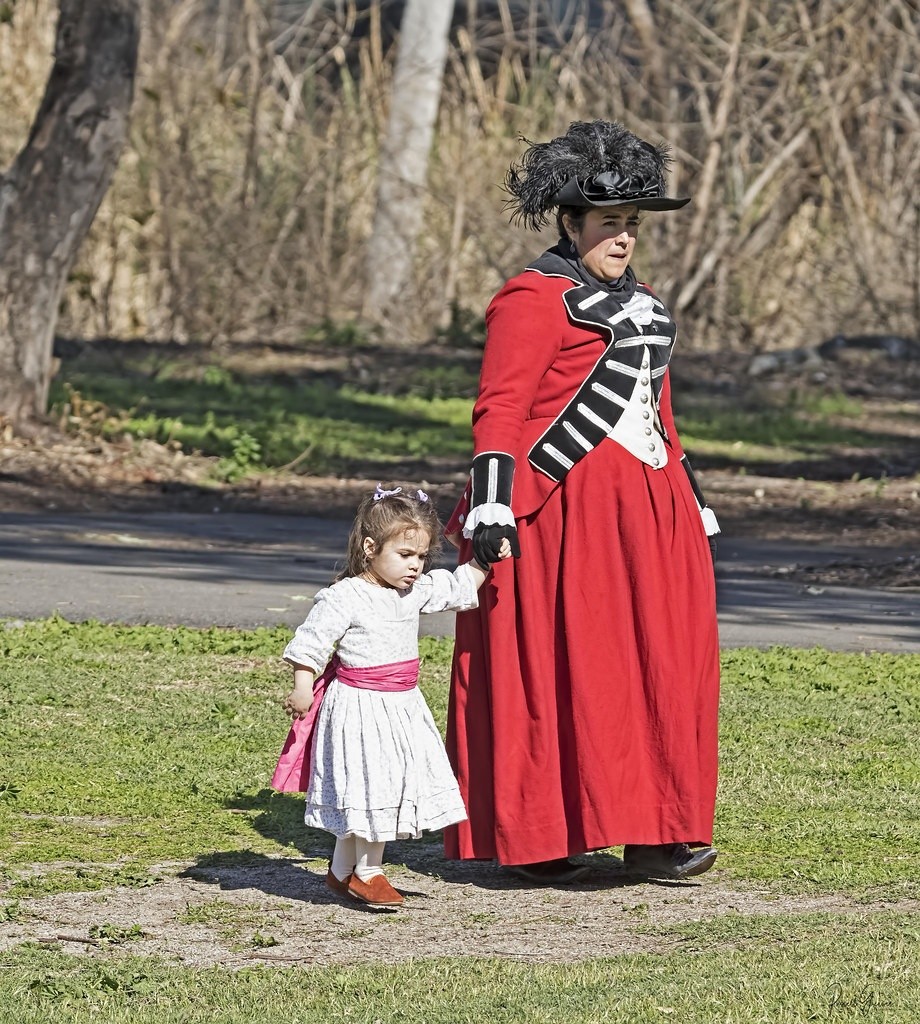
xmin=325 ymin=860 xmax=367 ymax=904
xmin=502 ymin=858 xmax=591 ymax=886
xmin=623 ymin=843 xmax=718 ymax=878
xmin=348 ymin=865 xmax=403 ymax=906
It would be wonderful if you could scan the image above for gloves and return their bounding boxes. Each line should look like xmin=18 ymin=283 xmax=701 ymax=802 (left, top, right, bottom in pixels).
xmin=707 ymin=535 xmax=717 ymax=567
xmin=471 ymin=521 xmax=522 ymax=572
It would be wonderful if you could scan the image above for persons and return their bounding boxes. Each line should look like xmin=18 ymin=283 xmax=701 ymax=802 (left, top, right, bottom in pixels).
xmin=438 ymin=122 xmax=720 ymax=886
xmin=281 ymin=483 xmax=513 ymax=908
